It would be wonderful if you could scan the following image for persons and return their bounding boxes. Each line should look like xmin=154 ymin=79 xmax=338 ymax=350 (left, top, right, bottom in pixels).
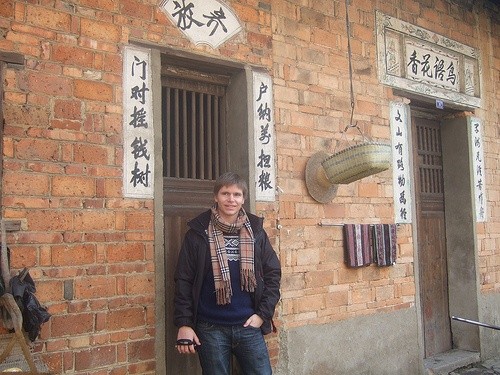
xmin=171 ymin=172 xmax=281 ymax=375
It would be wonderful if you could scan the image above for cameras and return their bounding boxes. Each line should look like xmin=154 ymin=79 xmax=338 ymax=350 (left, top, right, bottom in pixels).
xmin=193 ymin=340 xmax=202 ymax=352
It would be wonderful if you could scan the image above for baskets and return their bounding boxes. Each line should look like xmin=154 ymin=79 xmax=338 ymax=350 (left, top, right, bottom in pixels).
xmin=322 ymin=125 xmax=391 ymax=185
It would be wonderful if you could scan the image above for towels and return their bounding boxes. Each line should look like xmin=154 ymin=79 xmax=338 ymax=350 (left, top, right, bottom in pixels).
xmin=345 ymin=224 xmax=371 ymax=267
xmin=373 ymin=224 xmax=397 ymax=267
